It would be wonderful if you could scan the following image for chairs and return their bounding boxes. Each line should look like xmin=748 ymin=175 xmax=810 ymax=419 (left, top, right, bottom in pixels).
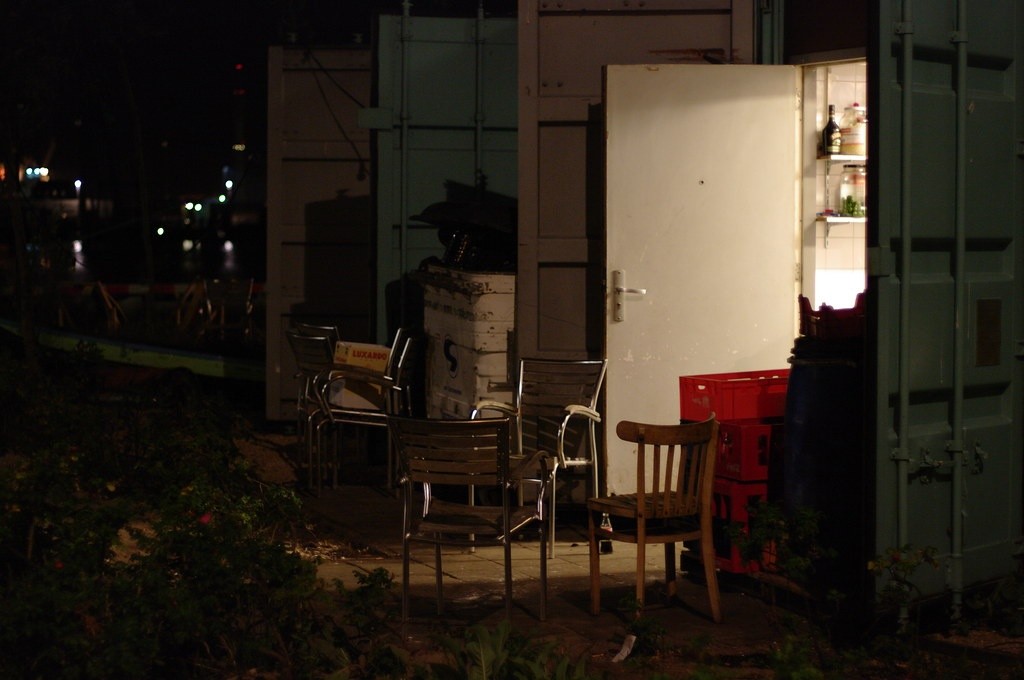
xmin=70 ymin=275 xmax=724 ymax=625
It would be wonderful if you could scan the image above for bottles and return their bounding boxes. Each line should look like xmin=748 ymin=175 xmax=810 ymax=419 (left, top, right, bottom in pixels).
xmin=822 ymin=103 xmax=867 ymax=218
xmin=600 ymin=513 xmax=614 ymax=553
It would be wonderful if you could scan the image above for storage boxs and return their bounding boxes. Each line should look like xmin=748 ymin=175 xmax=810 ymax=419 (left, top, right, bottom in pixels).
xmin=331 ymin=342 xmax=391 ymax=409
xmin=417 ymin=264 xmax=789 ymax=576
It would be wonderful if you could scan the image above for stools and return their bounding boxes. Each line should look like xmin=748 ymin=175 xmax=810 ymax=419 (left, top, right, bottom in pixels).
xmin=798 ymin=291 xmax=865 ymax=340
